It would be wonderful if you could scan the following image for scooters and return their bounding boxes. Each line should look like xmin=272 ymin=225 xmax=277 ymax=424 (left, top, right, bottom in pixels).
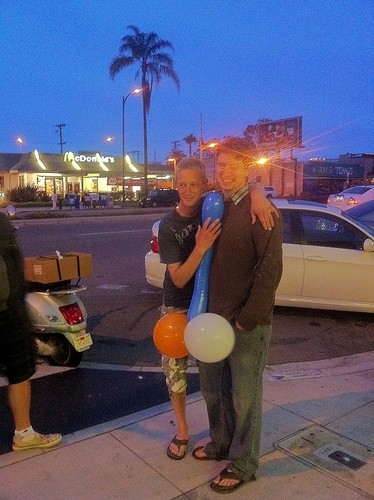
xmin=20 ymin=280 xmax=93 ymax=368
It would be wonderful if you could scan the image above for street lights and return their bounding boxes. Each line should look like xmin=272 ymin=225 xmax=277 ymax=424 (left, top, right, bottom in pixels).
xmin=17 ymin=137 xmax=24 ymax=153
xmin=199 ymin=141 xmax=218 ymax=161
xmin=168 ymin=158 xmax=177 ymax=171
xmin=121 ymin=87 xmax=141 ymax=208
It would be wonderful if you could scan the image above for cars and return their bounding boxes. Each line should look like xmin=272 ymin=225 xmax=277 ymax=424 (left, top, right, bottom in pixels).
xmin=264 ymin=186 xmax=277 ymax=198
xmin=327 ymin=185 xmax=374 ymax=218
xmin=144 ymin=197 xmax=374 ymax=313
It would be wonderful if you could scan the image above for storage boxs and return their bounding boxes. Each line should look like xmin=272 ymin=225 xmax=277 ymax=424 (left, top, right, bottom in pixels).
xmin=23 ymin=252 xmax=92 ymax=284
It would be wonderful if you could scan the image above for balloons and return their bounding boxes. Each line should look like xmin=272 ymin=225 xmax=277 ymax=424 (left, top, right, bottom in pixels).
xmin=186 ymin=192 xmax=225 ymax=321
xmin=183 ymin=312 xmax=236 ymax=364
xmin=152 ymin=311 xmax=189 ymax=359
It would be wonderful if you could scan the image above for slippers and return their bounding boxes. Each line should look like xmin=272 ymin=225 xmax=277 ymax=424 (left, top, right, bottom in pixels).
xmin=192 ymin=447 xmax=216 ymax=460
xmin=169 ymin=434 xmax=189 ymax=460
xmin=210 ymin=467 xmax=245 ymax=494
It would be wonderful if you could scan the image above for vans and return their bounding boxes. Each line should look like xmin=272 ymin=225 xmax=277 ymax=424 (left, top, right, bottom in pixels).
xmin=138 ymin=189 xmax=180 ymax=208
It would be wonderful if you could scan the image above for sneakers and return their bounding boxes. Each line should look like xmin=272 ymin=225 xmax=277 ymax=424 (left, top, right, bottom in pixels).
xmin=12 ymin=431 xmax=62 ymax=451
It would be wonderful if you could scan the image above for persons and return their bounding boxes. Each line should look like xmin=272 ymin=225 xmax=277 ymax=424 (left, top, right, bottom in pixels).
xmin=190 ymin=136 xmax=285 ymax=494
xmin=156 ymin=156 xmax=280 ymax=461
xmin=0 ymin=210 xmax=63 ymax=451
